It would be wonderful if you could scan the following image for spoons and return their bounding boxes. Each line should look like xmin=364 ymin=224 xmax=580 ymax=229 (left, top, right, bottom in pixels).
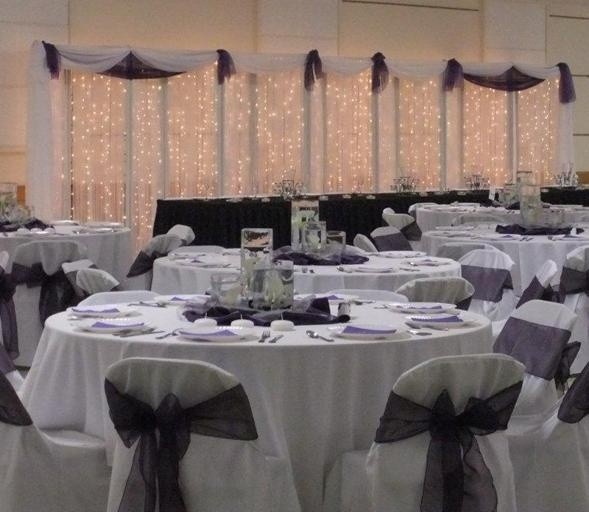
xmin=306 ymin=329 xmax=334 ymax=343
xmin=128 ymin=300 xmax=160 ymax=307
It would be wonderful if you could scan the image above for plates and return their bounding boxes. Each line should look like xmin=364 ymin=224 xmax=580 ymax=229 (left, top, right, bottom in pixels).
xmin=328 ymin=322 xmax=401 ymax=339
xmin=389 ymin=301 xmax=458 ymax=312
xmin=307 ymin=294 xmax=358 ymax=304
xmin=432 ymin=224 xmax=587 ymax=241
xmin=415 ymin=201 xmax=585 ymax=212
xmin=14 ymin=220 xmax=124 ymax=238
xmin=403 ymin=313 xmax=480 ymax=328
xmin=77 ymin=318 xmax=152 ymax=332
xmin=66 ymin=306 xmax=134 ymax=316
xmin=154 ymin=294 xmax=211 ymax=306
xmin=168 ymin=222 xmax=453 ymax=273
xmin=173 ymin=326 xmax=254 ymax=341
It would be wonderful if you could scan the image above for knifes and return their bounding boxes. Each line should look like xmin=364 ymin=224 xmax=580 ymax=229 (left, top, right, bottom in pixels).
xmin=406 ymin=330 xmax=432 ymax=336
xmin=269 ymin=334 xmax=284 ymax=343
xmin=155 ymin=327 xmax=181 ymax=339
xmin=120 ymin=329 xmax=166 ymax=338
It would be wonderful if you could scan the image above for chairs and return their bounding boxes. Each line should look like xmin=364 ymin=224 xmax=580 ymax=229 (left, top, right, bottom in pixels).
xmin=0 ymin=342 xmax=110 ymax=512
xmin=322 ymin=353 xmax=525 ymax=512
xmin=506 ymin=362 xmax=588 ymax=512
xmin=493 ymin=298 xmax=580 ymax=414
xmin=104 ymin=357 xmax=283 ymax=512
xmin=0 ymin=184 xmax=589 ymax=378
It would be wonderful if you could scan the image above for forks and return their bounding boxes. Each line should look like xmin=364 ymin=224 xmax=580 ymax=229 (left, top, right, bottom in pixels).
xmin=257 ymin=330 xmax=271 ymax=343
